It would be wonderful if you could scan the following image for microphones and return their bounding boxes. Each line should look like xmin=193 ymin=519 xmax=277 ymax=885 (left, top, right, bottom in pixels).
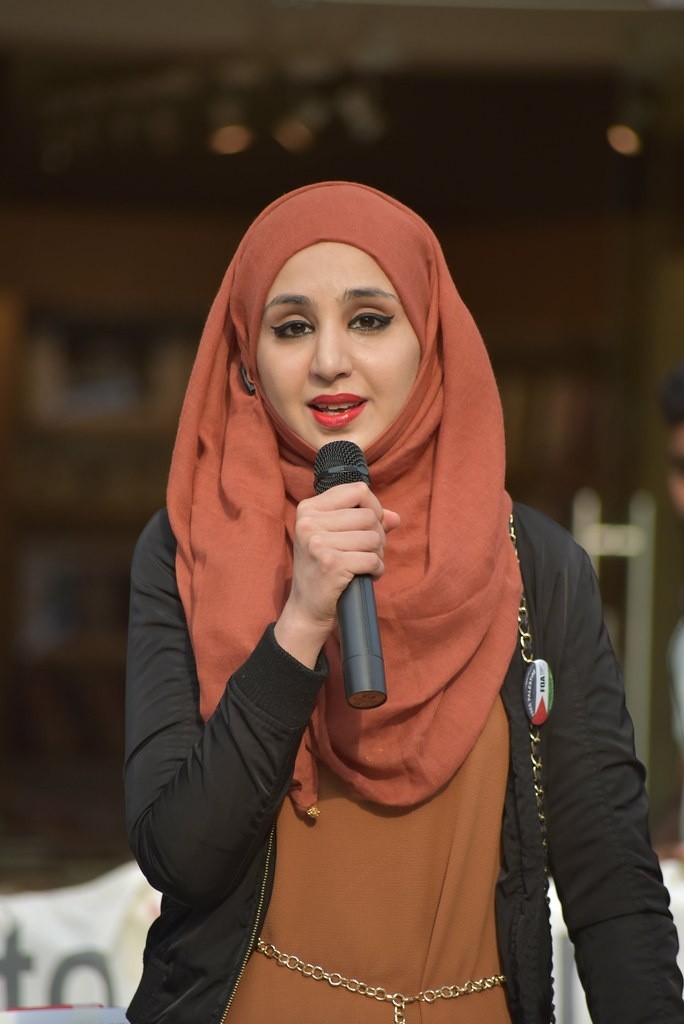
xmin=316 ymin=440 xmax=389 ymax=710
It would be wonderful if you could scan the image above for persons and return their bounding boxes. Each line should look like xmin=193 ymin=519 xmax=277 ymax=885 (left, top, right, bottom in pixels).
xmin=124 ymin=176 xmax=684 ymax=1023
